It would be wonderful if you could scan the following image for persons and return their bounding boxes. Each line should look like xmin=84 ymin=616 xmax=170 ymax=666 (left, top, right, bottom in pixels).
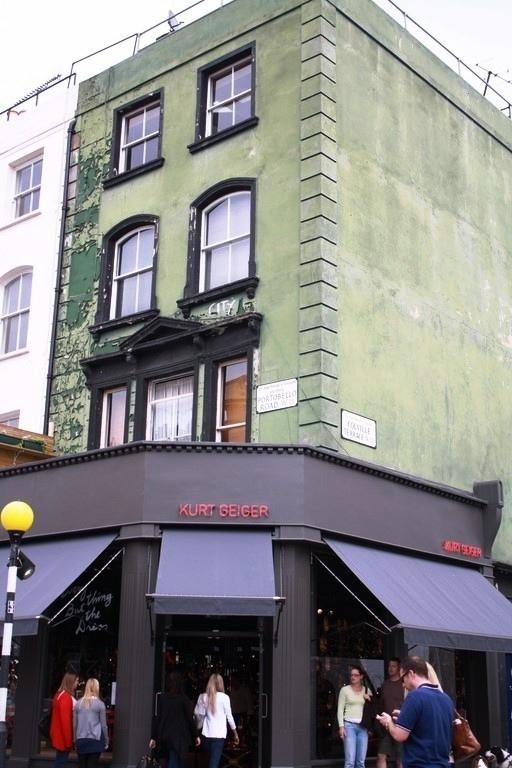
xmin=70 ymin=675 xmax=110 ymax=767
xmin=375 ymin=656 xmax=405 ymax=768
xmin=376 ymin=654 xmax=457 ymax=768
xmin=193 ymin=671 xmax=241 ymax=767
xmin=335 ymin=664 xmax=377 ymax=767
xmin=149 ymin=671 xmax=201 ymax=767
xmin=421 ymin=660 xmax=460 ymax=767
xmin=48 ymin=670 xmax=79 ymax=767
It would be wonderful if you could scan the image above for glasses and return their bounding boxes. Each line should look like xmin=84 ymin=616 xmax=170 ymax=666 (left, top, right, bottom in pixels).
xmin=400 ymin=672 xmax=409 ymax=682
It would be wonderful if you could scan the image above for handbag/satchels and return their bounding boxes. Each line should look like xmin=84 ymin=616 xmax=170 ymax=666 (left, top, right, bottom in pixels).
xmin=452 ymin=709 xmax=482 ymax=764
xmin=360 ymin=696 xmax=375 ymax=733
xmin=195 ymin=714 xmax=205 ymax=730
xmin=38 ymin=712 xmax=51 ymax=738
xmin=136 ymin=746 xmax=162 ymax=768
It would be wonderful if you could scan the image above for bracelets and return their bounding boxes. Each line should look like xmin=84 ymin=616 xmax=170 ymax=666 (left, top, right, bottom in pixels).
xmin=384 ymin=718 xmax=392 ymax=730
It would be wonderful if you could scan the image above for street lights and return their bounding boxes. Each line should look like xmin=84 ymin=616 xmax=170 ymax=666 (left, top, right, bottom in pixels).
xmin=0 ymin=497 xmax=36 ymax=768
